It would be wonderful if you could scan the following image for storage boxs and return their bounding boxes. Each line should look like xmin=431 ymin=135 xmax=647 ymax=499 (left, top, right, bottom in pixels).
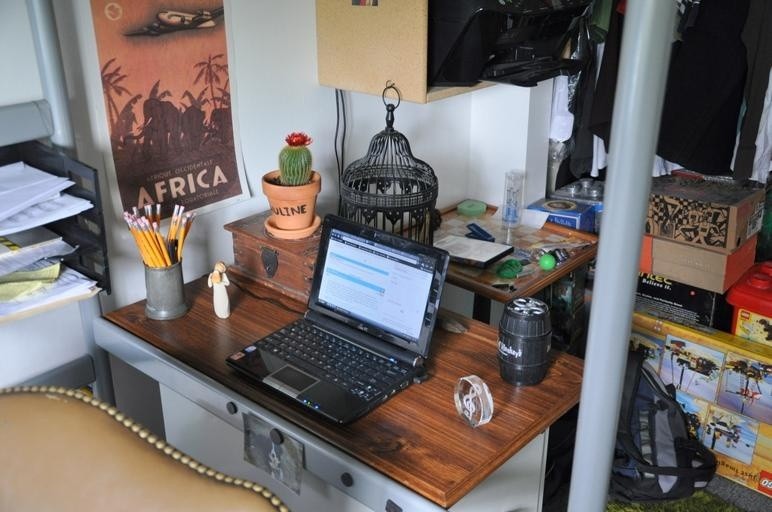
xmin=727 ymin=260 xmax=770 ymax=344
xmin=634 ymin=267 xmax=725 ymax=334
xmin=526 ymin=171 xmax=607 ymax=346
xmin=641 ymin=232 xmax=757 ymax=294
xmin=643 ymin=171 xmax=767 ymax=251
xmin=224 ymin=207 xmax=333 ymax=306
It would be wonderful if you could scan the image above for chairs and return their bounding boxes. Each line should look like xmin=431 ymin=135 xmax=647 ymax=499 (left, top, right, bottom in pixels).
xmin=2 ymin=383 xmax=282 ymax=510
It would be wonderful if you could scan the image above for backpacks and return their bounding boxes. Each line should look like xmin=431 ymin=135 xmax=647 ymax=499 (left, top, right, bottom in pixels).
xmin=607 ymin=339 xmax=719 ymax=506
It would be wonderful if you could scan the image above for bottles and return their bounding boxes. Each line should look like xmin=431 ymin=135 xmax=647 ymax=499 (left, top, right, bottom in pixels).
xmin=501 ymin=171 xmax=524 ymax=227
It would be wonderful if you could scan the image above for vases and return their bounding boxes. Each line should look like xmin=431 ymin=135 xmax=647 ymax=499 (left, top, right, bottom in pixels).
xmin=259 ymin=168 xmax=323 ymax=240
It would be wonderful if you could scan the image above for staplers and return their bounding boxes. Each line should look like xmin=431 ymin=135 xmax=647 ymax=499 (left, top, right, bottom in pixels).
xmin=465 ymin=222 xmax=495 ymax=242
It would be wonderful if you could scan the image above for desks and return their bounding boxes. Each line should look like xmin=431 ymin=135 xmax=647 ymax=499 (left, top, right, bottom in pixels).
xmin=90 ymin=271 xmax=584 ymax=510
xmin=394 ymin=197 xmax=598 ymax=327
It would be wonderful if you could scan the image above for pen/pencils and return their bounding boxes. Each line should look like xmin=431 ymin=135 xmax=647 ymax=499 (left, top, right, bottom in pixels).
xmin=123 ymin=203 xmax=197 ymax=267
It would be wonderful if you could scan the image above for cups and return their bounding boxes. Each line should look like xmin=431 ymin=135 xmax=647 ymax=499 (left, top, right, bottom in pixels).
xmin=141 ymin=258 xmax=188 ymax=320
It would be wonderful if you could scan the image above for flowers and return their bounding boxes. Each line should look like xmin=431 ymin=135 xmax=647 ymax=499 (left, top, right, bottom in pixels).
xmin=278 ymin=133 xmax=314 ymax=186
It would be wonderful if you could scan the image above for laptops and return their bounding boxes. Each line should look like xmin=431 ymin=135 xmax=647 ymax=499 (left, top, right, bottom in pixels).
xmin=225 ymin=213 xmax=450 ymax=427
